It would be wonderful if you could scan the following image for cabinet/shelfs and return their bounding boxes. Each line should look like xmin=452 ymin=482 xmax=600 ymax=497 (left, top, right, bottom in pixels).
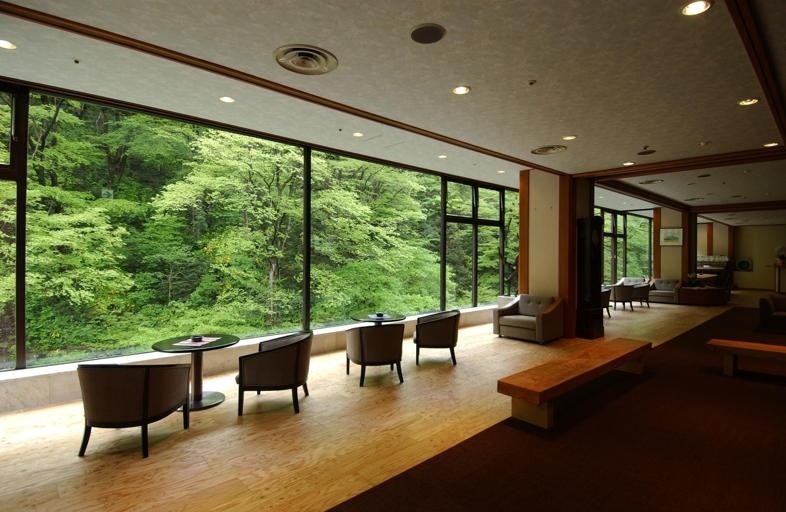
xmin=697 ymin=260 xmax=733 ymax=289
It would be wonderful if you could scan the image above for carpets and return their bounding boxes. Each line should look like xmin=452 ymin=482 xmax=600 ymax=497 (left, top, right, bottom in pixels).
xmin=325 ymin=305 xmax=785 ymax=512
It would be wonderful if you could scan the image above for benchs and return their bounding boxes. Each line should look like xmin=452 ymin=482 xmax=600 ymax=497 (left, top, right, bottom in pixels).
xmin=495 ymin=336 xmax=653 ymax=429
xmin=707 ymin=339 xmax=786 ymax=377
xmin=680 ymin=285 xmax=730 ymax=306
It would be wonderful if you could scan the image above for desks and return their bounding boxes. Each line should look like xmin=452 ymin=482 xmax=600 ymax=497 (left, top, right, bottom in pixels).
xmin=153 ymin=334 xmax=240 ymax=410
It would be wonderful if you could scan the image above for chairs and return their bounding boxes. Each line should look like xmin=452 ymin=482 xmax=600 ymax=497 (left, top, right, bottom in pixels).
xmin=235 ymin=329 xmax=313 ymax=414
xmin=345 ymin=324 xmax=404 ymax=387
xmin=601 ymin=277 xmax=682 ymax=319
xmin=413 ymin=309 xmax=460 ymax=365
xmin=492 ymin=294 xmax=564 ymax=345
xmin=758 ymin=292 xmax=786 ymax=333
xmin=76 ymin=364 xmax=191 ymax=458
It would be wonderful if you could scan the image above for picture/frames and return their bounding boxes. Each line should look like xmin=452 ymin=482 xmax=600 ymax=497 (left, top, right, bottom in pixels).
xmin=658 ymin=228 xmax=684 ymax=247
